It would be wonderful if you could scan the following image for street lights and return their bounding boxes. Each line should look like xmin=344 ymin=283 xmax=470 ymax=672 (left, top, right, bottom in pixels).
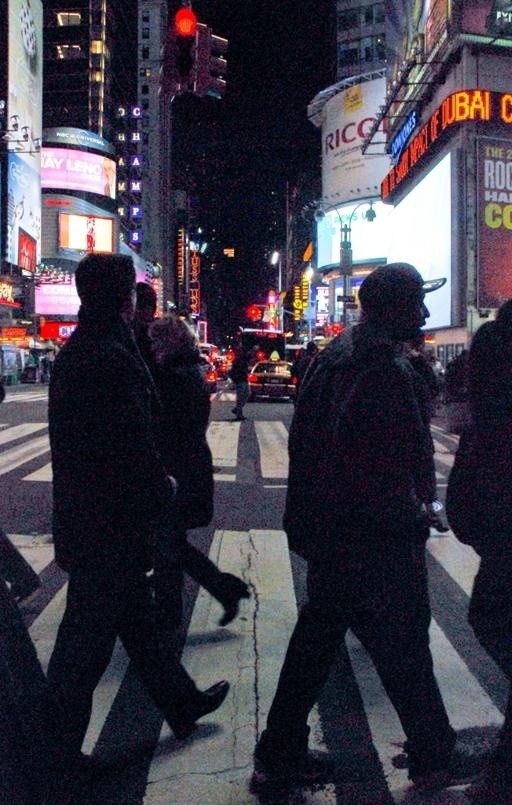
xmin=269 ymin=250 xmax=284 ymax=334
xmin=311 ymin=198 xmax=379 ymax=327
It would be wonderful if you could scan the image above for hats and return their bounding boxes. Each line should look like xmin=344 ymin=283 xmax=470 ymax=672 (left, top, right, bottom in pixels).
xmin=359 ymin=263 xmax=446 ymax=311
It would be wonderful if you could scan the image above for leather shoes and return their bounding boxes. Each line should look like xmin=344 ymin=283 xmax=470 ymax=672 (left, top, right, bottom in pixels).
xmin=172 ymin=681 xmax=229 ymax=737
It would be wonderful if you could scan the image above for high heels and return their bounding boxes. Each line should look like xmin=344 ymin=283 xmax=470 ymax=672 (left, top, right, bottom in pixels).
xmin=218 ymin=591 xmax=250 ymax=626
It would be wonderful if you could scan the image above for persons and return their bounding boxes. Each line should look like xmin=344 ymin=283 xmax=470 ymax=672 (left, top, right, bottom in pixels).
xmin=247 ymin=265 xmax=495 ymax=793
xmin=132 ymin=282 xmax=156 ymax=362
xmin=84 ymin=218 xmax=97 ymax=250
xmin=48 ymin=253 xmax=233 ymax=768
xmin=102 ymin=157 xmax=115 ymax=196
xmin=198 ymin=334 xmax=475 ymax=420
xmin=0 ymin=528 xmax=116 ymax=805
xmin=139 ymin=305 xmax=254 ymax=630
xmin=446 ymin=294 xmax=512 ymax=805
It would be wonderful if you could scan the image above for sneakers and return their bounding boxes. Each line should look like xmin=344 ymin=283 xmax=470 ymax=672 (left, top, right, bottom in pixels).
xmin=250 ymin=748 xmax=348 ymax=795
xmin=424 ymin=513 xmax=449 ymax=533
xmin=412 ymin=741 xmax=497 ymax=794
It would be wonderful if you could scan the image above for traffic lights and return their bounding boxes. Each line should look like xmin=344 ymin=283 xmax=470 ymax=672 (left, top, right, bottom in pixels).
xmin=189 ymin=22 xmax=231 ymax=103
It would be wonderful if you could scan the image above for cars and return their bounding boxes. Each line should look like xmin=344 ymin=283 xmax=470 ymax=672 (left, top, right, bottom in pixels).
xmin=196 ymin=328 xmax=308 ymax=402
